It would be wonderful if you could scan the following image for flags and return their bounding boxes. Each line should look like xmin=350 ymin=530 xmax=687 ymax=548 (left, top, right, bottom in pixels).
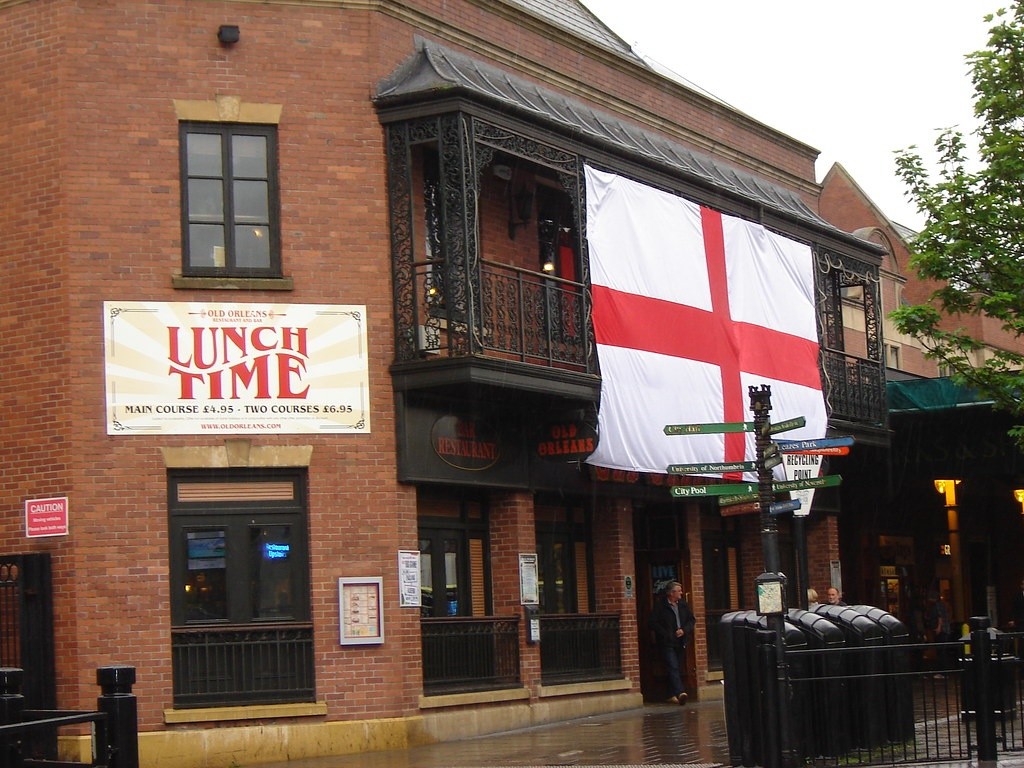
xmin=582 ymin=163 xmax=828 ymax=482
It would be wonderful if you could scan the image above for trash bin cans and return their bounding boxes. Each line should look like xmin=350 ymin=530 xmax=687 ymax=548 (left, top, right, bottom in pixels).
xmin=719 ymin=602 xmax=916 ymax=768
xmin=957 ymin=627 xmax=1017 ymax=720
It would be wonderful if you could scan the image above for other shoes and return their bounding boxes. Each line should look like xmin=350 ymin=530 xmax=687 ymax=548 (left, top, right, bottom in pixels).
xmin=679 ymin=692 xmax=687 ymax=705
xmin=666 ymin=697 xmax=678 ymax=703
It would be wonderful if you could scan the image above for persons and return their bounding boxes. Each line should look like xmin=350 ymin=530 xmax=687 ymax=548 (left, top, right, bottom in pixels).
xmin=654 ymin=582 xmax=696 ymax=706
xmin=907 ymin=590 xmax=951 ymax=680
xmin=807 ymin=587 xmax=848 ymax=609
xmin=1007 ymin=576 xmax=1024 ymax=674
